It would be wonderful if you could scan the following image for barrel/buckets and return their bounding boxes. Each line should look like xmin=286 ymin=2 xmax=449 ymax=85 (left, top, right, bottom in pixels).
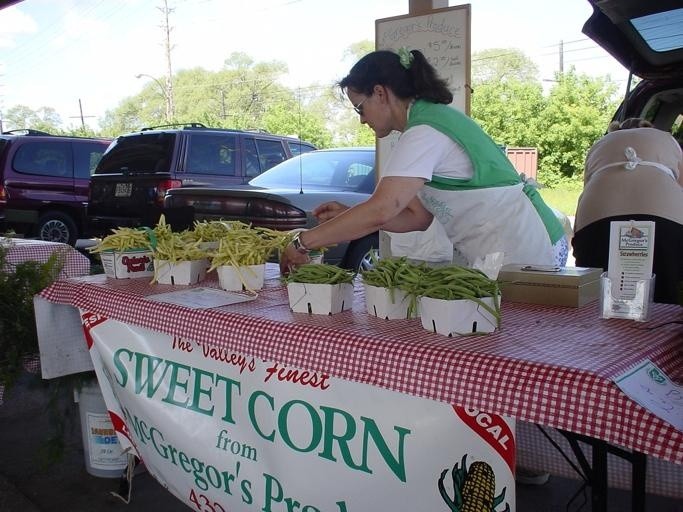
xmin=69 ymin=378 xmax=147 ymax=479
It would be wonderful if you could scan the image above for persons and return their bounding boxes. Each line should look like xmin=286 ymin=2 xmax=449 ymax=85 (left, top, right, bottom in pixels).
xmin=571 ymin=118 xmax=683 ymax=306
xmin=280 ymin=45 xmax=568 ymax=280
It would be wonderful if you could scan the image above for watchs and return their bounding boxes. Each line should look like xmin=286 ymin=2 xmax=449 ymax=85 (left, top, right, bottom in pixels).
xmin=291 ymin=232 xmax=312 ymax=254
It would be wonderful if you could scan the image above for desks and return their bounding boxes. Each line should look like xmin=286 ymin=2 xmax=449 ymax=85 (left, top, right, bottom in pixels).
xmin=0 ymin=234 xmax=90 ymax=407
xmin=34 ymin=265 xmax=683 ymax=512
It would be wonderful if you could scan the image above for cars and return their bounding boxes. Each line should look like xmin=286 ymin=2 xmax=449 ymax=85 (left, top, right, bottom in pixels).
xmin=164 ymin=148 xmax=378 ymax=275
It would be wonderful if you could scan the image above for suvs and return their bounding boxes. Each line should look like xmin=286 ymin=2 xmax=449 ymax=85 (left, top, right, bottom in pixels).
xmin=581 ymin=1 xmax=683 ymax=138
xmin=87 ymin=124 xmax=317 ymax=238
xmin=0 ymin=129 xmax=113 ymax=248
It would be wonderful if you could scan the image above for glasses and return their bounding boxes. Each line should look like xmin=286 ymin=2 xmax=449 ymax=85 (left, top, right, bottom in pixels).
xmin=353 ymin=96 xmax=367 ymax=114
